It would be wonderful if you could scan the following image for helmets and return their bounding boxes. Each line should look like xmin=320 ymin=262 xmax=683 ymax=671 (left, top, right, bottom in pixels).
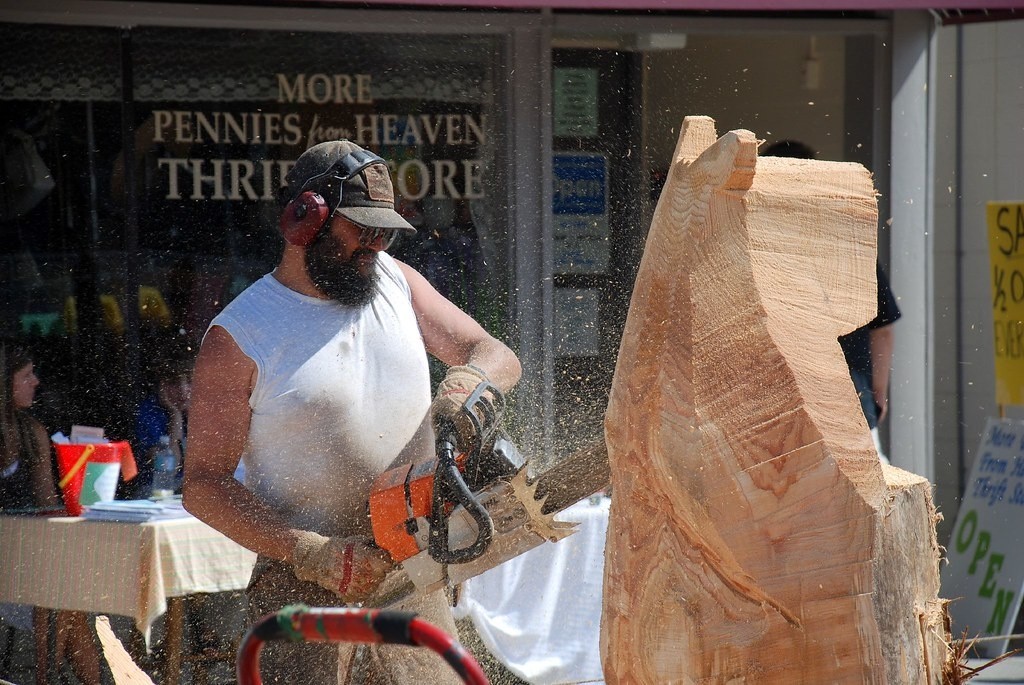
xmin=142 ymin=328 xmax=202 ymax=390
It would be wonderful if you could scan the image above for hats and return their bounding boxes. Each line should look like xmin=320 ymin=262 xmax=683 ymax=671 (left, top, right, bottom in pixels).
xmin=277 ymin=140 xmax=417 ymax=237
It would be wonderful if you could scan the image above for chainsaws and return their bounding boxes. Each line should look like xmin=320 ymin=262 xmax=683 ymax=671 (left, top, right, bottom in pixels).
xmin=350 ymin=421 xmax=612 ymax=610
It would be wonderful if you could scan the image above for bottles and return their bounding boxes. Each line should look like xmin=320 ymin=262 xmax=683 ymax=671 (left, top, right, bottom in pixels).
xmin=151 ymin=435 xmax=176 ymax=498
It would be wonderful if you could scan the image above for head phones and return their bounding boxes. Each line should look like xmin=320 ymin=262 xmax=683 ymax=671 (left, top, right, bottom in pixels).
xmin=278 ymin=149 xmax=393 ymax=246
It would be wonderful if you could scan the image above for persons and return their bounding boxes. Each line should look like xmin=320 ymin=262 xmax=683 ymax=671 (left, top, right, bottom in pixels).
xmin=0 ymin=342 xmax=57 ymax=505
xmin=182 ymin=140 xmax=522 ymax=684
xmin=135 ymin=360 xmax=192 ymax=493
xmin=764 ymin=139 xmax=901 ymax=431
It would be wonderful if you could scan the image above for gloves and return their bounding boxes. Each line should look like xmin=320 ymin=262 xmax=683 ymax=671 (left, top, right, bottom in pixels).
xmin=294 ymin=531 xmax=392 ymax=602
xmin=432 ymin=366 xmax=496 ymax=450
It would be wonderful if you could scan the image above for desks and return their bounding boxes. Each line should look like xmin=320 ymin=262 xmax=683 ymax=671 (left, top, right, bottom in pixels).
xmin=0 ymin=502 xmax=258 ymax=685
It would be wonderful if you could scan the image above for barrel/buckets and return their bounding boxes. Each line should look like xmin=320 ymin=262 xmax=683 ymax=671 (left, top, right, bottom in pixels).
xmin=51 ymin=440 xmax=128 ymax=517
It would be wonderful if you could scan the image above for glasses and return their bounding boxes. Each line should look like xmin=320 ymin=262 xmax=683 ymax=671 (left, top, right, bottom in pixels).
xmin=335 ymin=217 xmax=398 ymax=251
